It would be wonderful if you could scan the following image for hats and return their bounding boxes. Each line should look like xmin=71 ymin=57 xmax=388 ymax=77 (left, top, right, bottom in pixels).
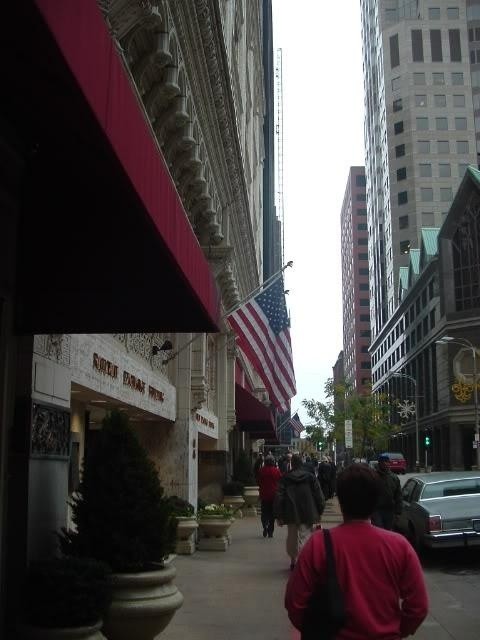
xmin=321 ymin=456 xmax=328 ymax=462
xmin=378 ymin=453 xmax=389 ymax=462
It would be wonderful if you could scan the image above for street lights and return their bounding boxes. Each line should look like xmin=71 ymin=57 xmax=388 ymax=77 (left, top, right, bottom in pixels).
xmin=435 ymin=337 xmax=480 ymax=471
xmin=388 ymin=370 xmax=420 ymax=464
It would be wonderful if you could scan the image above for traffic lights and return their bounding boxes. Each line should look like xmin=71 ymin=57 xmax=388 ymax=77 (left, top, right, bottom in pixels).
xmin=317 ymin=441 xmax=323 ymax=451
xmin=422 ymin=431 xmax=431 ymax=450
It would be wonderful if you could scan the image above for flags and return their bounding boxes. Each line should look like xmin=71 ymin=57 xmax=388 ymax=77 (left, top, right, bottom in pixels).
xmin=224 ymin=272 xmax=296 ymax=408
xmin=289 ymin=413 xmax=305 ymax=433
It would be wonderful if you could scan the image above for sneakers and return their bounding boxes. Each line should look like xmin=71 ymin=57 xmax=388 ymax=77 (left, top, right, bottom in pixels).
xmin=290 ymin=559 xmax=296 ymax=569
xmin=262 ymin=527 xmax=274 ymax=538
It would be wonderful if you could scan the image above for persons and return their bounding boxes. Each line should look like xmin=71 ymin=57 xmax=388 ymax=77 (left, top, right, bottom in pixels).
xmin=284 ymin=462 xmax=429 ymax=640
xmin=369 ymin=456 xmax=404 ymax=531
xmin=253 ymin=452 xmax=336 ymax=502
xmin=256 ymin=455 xmax=281 ymax=539
xmin=273 ymin=453 xmax=325 ymax=571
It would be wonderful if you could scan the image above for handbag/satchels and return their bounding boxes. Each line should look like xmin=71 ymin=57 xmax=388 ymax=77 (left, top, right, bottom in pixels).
xmin=300 ymin=574 xmax=346 ymax=640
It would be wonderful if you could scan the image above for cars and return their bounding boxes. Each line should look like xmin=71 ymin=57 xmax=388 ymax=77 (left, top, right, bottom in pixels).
xmin=334 ymin=453 xmax=407 ymax=472
xmin=394 ymin=471 xmax=480 ymax=553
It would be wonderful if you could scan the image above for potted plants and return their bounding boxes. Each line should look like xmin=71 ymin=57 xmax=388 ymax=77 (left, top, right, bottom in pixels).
xmin=199 ymin=504 xmax=236 ymax=551
xmin=241 ymin=480 xmax=261 ymax=515
xmin=72 ymin=405 xmax=184 ymax=640
xmin=23 ymin=540 xmax=110 ymax=640
xmin=165 ymin=496 xmax=201 ymax=555
xmin=220 ymin=481 xmax=246 ymax=520
